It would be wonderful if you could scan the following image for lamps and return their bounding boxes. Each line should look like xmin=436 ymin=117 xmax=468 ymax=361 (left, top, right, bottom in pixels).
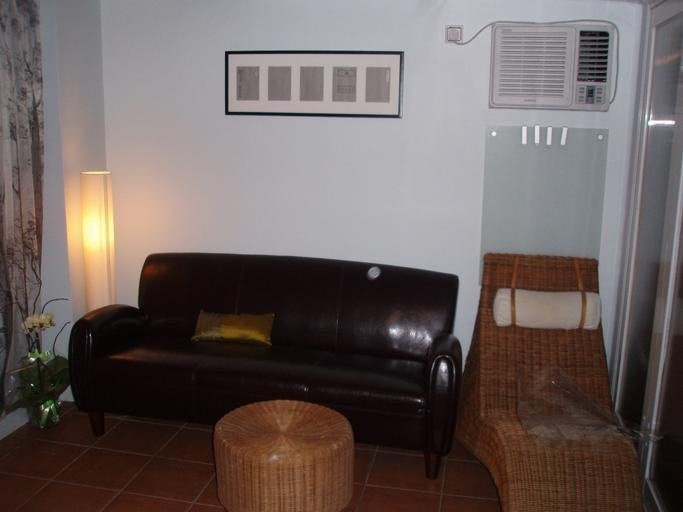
xmin=79 ymin=171 xmax=117 ymax=311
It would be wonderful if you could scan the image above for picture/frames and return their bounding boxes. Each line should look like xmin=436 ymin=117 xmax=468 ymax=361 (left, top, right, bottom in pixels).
xmin=225 ymin=50 xmax=404 ymax=118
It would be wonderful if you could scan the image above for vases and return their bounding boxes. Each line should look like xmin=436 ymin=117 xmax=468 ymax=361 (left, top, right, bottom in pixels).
xmin=0 ymin=355 xmax=70 ymax=430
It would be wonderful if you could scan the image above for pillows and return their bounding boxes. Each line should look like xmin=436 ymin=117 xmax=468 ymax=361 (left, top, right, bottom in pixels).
xmin=189 ymin=308 xmax=276 ymax=348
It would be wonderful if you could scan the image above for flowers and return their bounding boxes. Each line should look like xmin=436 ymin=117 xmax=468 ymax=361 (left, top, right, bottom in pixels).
xmin=17 ymin=311 xmax=55 ymax=393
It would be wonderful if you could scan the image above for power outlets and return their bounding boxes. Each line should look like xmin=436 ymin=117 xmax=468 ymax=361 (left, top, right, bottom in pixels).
xmin=447 ymin=27 xmax=461 ymax=42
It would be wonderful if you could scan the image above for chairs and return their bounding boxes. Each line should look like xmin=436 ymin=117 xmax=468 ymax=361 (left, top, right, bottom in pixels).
xmin=452 ymin=251 xmax=646 ymax=512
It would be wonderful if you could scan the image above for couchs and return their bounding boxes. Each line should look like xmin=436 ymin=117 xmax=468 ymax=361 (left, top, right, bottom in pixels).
xmin=64 ymin=252 xmax=462 ymax=481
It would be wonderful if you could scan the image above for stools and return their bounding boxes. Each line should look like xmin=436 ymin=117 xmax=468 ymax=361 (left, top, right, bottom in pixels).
xmin=213 ymin=399 xmax=355 ymax=512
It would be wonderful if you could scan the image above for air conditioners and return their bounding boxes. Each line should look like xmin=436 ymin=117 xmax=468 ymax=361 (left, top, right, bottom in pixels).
xmin=488 ymin=21 xmax=614 ymax=113
